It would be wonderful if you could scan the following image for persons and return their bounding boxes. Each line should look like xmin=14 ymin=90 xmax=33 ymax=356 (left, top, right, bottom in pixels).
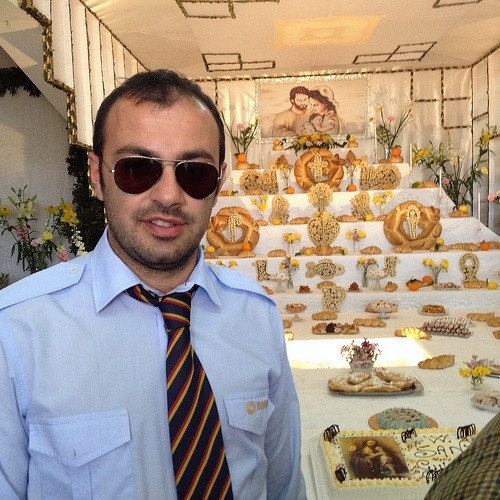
xmin=424 ymin=412 xmax=500 ymax=500
xmin=1 ymin=69 xmax=309 ymax=500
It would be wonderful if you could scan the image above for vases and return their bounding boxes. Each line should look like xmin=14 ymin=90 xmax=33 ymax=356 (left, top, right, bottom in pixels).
xmin=348 ymin=142 xmax=358 ymax=148
xmin=288 ymin=244 xmax=293 ymax=257
xmin=258 ymin=209 xmax=265 ymax=219
xmin=383 ymin=145 xmax=393 ymax=160
xmin=284 ymin=177 xmax=289 ymax=188
xmin=363 ymin=274 xmax=368 ymax=287
xmin=353 ymin=240 xmax=359 ymax=255
xmin=433 ymin=275 xmax=440 ymax=287
xmin=286 ymin=276 xmax=293 ymax=289
xmin=349 ymin=360 xmax=374 ymax=373
xmin=348 ymin=175 xmax=354 ymax=184
xmin=274 ymin=144 xmax=283 ymax=151
xmin=471 ymin=376 xmax=484 ymax=390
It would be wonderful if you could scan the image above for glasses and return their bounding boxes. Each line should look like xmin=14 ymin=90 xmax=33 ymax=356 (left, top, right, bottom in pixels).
xmin=99 ymin=156 xmax=223 ymax=199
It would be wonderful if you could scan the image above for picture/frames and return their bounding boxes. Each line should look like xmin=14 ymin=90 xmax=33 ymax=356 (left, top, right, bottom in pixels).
xmin=251 ymin=74 xmax=377 ymax=144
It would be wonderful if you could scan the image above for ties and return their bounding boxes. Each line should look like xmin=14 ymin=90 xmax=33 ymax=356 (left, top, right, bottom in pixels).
xmin=122 ymin=284 xmax=234 ymax=500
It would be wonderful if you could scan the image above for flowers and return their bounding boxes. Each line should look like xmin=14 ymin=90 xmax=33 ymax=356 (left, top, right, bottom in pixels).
xmin=217 ymin=108 xmax=261 ymax=154
xmin=204 ymin=231 xmax=449 ymax=274
xmin=341 ymin=339 xmax=380 ymax=362
xmin=250 ymin=194 xmax=269 ymax=210
xmin=272 ymin=132 xmax=356 ymax=150
xmin=459 ymin=355 xmax=494 ymax=379
xmin=277 ymin=158 xmax=360 ymax=176
xmin=374 ymin=97 xmax=500 ymax=212
xmin=0 ymin=184 xmax=85 ymax=274
